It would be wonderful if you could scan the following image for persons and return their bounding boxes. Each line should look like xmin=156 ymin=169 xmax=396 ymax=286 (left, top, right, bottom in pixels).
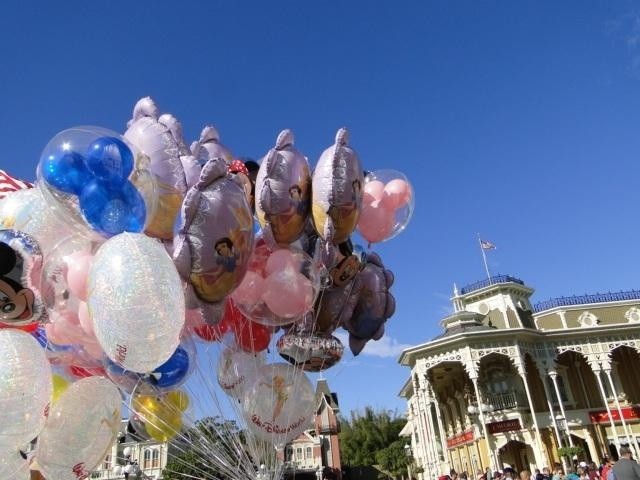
xmin=438 ymin=445 xmax=640 ymax=480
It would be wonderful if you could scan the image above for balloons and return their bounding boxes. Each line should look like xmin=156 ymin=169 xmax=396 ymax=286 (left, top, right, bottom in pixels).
xmin=1 ymin=169 xmax=122 ymax=480
xmin=36 ymin=96 xmax=414 ymax=450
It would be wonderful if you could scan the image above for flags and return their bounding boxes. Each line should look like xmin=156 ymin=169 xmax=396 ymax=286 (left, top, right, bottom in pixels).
xmin=480 ymin=240 xmax=496 ymax=250
